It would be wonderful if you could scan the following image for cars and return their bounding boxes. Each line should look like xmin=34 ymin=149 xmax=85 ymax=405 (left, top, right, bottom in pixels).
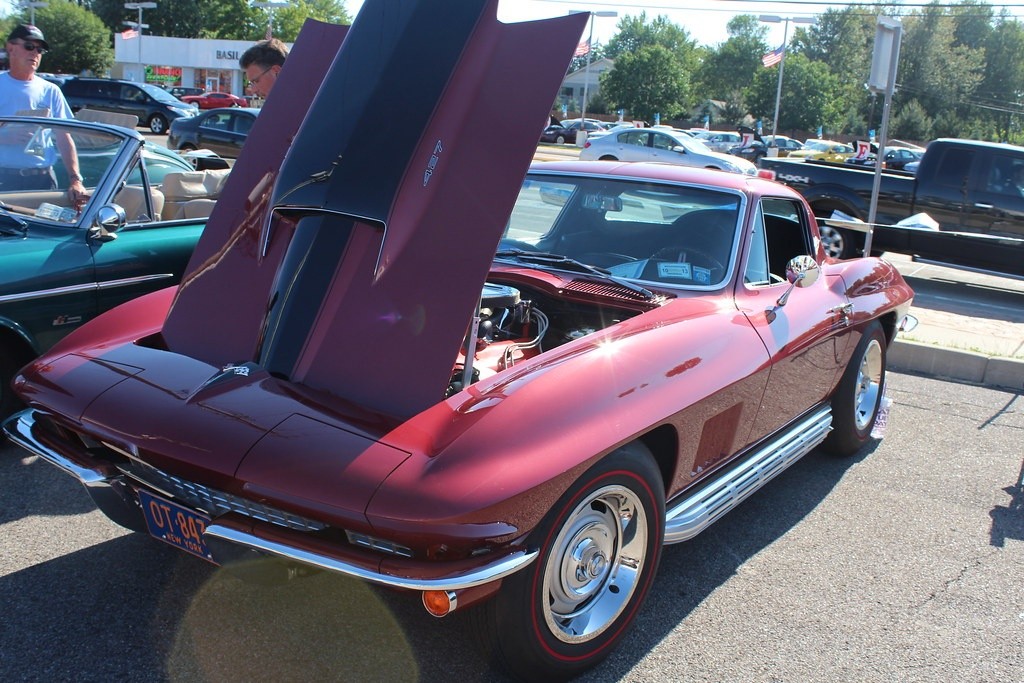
xmin=577 ymin=128 xmax=758 ymax=175
xmin=541 ymin=118 xmax=927 ymax=171
xmin=167 ymin=107 xmax=262 ymax=171
xmin=35 ymin=71 xmax=247 ymax=135
xmin=2 ymin=0 xmax=915 ymax=683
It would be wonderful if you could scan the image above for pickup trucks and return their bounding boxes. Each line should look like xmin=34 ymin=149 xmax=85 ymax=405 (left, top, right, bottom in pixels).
xmin=760 ymin=138 xmax=1024 ymax=281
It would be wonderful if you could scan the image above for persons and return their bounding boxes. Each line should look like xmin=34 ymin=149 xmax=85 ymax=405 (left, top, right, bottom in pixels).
xmin=0 ymin=24 xmax=88 ymax=211
xmin=239 ymin=40 xmax=291 ymax=101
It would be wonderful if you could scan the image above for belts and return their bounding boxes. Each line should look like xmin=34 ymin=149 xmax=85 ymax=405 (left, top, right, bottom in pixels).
xmin=0 ymin=168 xmax=47 ymax=177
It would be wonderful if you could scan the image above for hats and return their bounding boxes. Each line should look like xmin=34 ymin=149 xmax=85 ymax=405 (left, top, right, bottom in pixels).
xmin=8 ymin=24 xmax=48 ymax=49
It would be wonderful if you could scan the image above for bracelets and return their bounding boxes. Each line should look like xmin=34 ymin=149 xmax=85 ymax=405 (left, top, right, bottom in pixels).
xmin=71 ymin=173 xmax=84 ymax=182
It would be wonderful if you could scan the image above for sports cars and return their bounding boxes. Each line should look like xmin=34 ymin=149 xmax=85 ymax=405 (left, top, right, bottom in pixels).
xmin=0 ymin=116 xmax=230 ymax=451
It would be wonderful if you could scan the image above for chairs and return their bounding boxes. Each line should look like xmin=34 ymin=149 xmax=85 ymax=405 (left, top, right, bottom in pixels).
xmin=666 ymin=207 xmax=807 ymax=280
xmin=114 ymin=185 xmax=165 ymax=221
xmin=174 ymin=198 xmax=217 ymax=219
xmin=209 ymin=115 xmax=219 ymax=127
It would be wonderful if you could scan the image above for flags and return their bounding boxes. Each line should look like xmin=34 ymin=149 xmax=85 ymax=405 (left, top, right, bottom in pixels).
xmin=761 ymin=43 xmax=783 ymax=67
xmin=575 ymin=36 xmax=591 ymax=57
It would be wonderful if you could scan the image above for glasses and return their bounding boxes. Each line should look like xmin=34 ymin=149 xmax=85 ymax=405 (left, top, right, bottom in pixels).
xmin=248 ymin=67 xmax=272 ymax=87
xmin=12 ymin=42 xmax=46 ymax=54
